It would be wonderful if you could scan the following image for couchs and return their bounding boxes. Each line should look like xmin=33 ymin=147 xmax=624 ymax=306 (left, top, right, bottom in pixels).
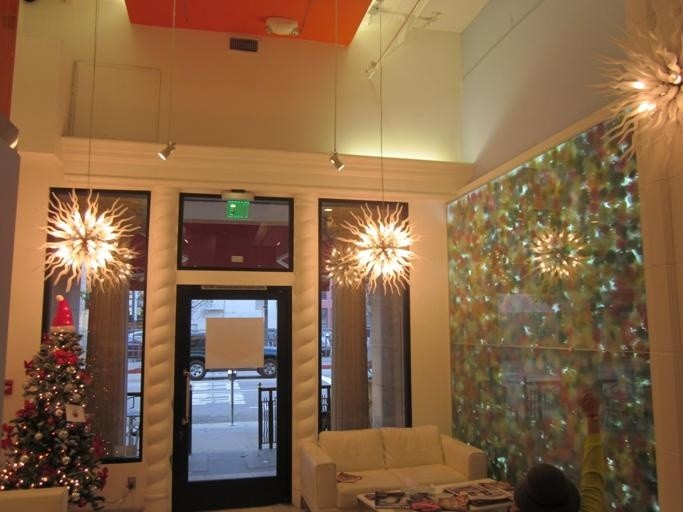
xmin=298 ymin=422 xmax=494 ymax=512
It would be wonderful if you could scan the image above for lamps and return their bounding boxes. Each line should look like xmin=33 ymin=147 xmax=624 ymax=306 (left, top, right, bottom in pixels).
xmin=36 ymin=0 xmax=143 ymax=296
xmin=155 ymin=0 xmax=178 ymax=161
xmin=326 ymin=244 xmax=364 ymax=293
xmin=329 ymin=1 xmax=423 ymax=298
xmin=582 ymin=10 xmax=681 ymax=169
xmin=525 ymin=221 xmax=594 ymax=284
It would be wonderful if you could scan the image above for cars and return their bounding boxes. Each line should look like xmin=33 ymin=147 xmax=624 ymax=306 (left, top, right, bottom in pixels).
xmin=128 ymin=328 xmax=332 ymax=380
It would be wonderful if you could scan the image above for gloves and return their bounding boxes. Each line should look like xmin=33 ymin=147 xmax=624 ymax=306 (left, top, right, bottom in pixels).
xmin=576 ymin=385 xmax=600 ymax=433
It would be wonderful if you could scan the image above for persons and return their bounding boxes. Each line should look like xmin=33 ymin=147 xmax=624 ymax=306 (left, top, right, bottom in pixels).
xmin=511 ymin=385 xmax=606 ymax=511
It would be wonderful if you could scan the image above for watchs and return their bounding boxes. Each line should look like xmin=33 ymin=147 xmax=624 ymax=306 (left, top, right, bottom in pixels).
xmin=586 ymin=414 xmax=601 ymax=421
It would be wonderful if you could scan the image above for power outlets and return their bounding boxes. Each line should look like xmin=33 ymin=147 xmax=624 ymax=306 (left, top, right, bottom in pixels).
xmin=126 ymin=476 xmax=135 ymax=490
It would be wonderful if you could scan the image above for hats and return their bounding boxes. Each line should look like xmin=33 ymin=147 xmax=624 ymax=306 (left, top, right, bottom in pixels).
xmin=513 ymin=463 xmax=580 ymax=512
